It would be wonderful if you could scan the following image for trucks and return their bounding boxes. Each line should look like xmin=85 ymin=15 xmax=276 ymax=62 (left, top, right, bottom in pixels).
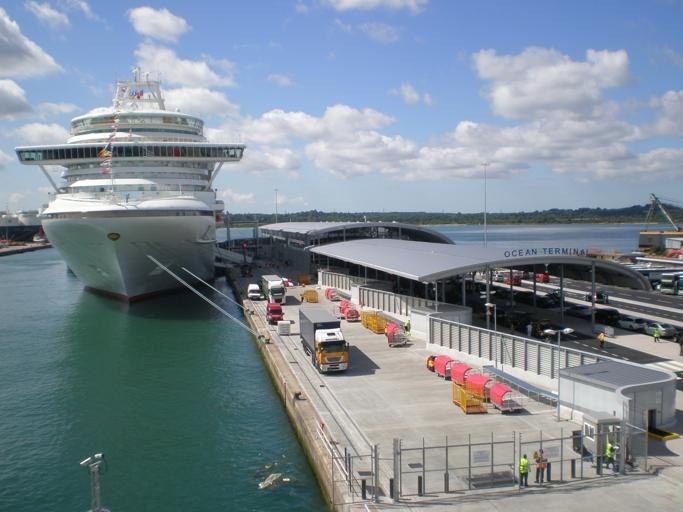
xmin=299 ymin=308 xmax=348 ymax=373
xmin=260 ymin=275 xmax=286 ymax=305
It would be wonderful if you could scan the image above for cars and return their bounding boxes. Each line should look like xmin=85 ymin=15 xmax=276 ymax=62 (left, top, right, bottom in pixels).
xmin=490 ymin=270 xmax=565 ymax=308
xmin=480 ymin=308 xmax=565 ymax=343
xmin=582 ymin=289 xmax=683 ymax=344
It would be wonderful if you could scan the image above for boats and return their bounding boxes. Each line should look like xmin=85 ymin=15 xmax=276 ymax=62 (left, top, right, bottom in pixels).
xmin=14 ymin=66 xmax=246 ymax=303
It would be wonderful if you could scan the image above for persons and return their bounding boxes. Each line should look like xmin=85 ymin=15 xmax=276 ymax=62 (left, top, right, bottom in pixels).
xmin=598 ymin=331 xmax=605 ymax=348
xmin=606 ymin=439 xmax=615 ymax=469
xmin=519 ymin=454 xmax=531 ymax=487
xmin=654 ymin=329 xmax=660 ymax=342
xmin=533 ymin=449 xmax=546 ymax=483
xmin=405 ymin=320 xmax=411 ymax=336
xmin=679 ymin=335 xmax=683 ymax=355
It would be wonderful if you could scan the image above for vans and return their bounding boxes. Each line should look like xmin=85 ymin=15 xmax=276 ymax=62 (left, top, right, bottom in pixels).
xmin=266 ymin=303 xmax=285 ymax=325
xmin=247 ymin=283 xmax=261 ymax=300
